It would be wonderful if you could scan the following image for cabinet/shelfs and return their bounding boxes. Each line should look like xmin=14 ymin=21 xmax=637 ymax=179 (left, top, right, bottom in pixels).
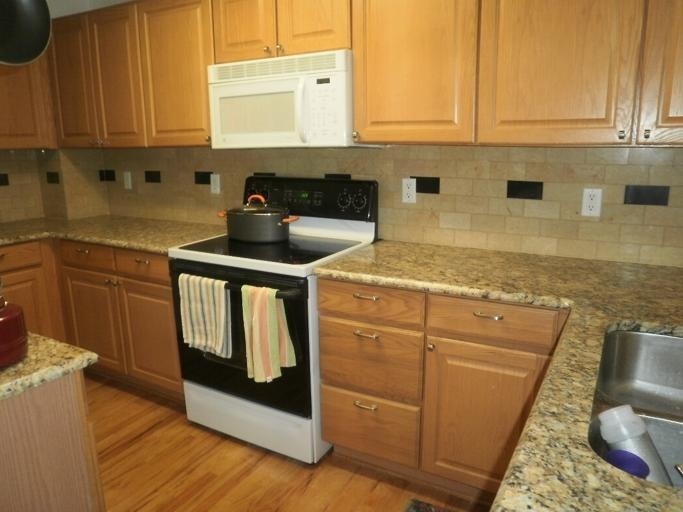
xmin=48 ymin=1 xmax=147 ymax=151
xmin=421 ymin=291 xmax=572 ymax=507
xmin=52 ymin=237 xmax=186 ymax=408
xmin=0 ymin=39 xmax=59 ymax=153
xmin=210 ymin=1 xmax=353 ymax=67
xmin=319 ymin=277 xmax=429 ymax=494
xmin=349 ymin=1 xmax=482 ymax=148
xmin=1 ymin=363 xmax=107 ymax=512
xmin=139 ymin=1 xmax=214 ymax=151
xmin=475 ymin=1 xmax=683 ymax=151
xmin=0 ymin=239 xmax=70 ymax=346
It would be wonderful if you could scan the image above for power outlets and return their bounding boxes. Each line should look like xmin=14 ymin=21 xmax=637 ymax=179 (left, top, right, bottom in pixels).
xmin=401 ymin=177 xmax=419 ymax=207
xmin=580 ymin=187 xmax=605 ymax=219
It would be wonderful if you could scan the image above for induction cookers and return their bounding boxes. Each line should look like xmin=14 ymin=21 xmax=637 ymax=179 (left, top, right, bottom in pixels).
xmin=178 ymin=232 xmax=363 ymax=263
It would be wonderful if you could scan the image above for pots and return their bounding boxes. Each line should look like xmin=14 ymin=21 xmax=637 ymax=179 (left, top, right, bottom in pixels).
xmin=215 ymin=194 xmax=298 ymax=243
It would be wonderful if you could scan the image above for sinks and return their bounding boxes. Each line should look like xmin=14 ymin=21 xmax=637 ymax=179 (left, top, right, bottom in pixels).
xmin=588 ymin=409 xmax=683 ymax=489
xmin=596 ymin=328 xmax=683 ymax=418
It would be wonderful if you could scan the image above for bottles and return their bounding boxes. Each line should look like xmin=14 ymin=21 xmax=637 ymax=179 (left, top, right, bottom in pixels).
xmin=598 ymin=403 xmax=673 ymax=488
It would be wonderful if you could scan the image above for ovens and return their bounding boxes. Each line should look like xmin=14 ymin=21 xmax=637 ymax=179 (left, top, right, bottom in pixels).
xmin=168 ymin=256 xmax=312 ymax=466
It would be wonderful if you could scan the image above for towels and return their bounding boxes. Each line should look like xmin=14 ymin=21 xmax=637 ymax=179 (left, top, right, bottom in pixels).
xmin=177 ymin=273 xmax=233 ymax=361
xmin=241 ymin=284 xmax=297 ymax=385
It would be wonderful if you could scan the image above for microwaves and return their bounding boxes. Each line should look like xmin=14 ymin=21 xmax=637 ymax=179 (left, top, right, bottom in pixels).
xmin=205 ymin=49 xmax=350 ymax=147
